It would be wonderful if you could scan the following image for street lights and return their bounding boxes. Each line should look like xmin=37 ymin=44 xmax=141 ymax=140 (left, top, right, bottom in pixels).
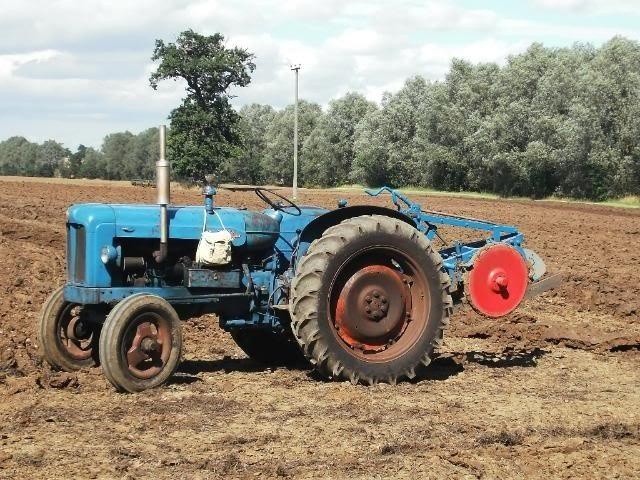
xmin=287 ymin=63 xmax=304 ymax=201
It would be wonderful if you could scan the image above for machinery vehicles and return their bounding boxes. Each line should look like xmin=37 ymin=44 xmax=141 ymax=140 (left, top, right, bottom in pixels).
xmin=37 ymin=119 xmax=563 ymax=395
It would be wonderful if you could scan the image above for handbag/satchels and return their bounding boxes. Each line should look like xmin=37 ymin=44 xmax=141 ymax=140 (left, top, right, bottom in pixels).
xmin=194 ymin=227 xmax=234 ymax=267
